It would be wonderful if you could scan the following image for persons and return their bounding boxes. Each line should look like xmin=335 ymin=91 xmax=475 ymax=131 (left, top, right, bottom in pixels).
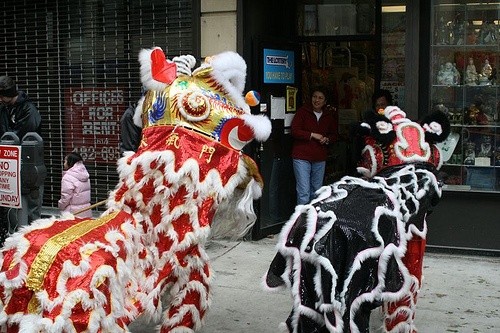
xmin=372 ymin=88 xmax=392 ymax=116
xmin=0 ymin=75 xmax=42 ymax=139
xmin=118 ymin=103 xmax=141 ymax=157
xmin=290 ymin=87 xmax=339 ymax=204
xmin=332 ymin=71 xmax=357 ymax=109
xmin=57 ymin=151 xmax=93 ymax=217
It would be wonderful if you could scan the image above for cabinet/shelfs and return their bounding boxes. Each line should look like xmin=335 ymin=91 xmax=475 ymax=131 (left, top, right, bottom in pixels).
xmin=404 ymin=0 xmax=500 ymax=257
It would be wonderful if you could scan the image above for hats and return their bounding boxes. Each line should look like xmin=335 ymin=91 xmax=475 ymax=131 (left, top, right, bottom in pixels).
xmin=0 ymin=75 xmax=17 ymax=96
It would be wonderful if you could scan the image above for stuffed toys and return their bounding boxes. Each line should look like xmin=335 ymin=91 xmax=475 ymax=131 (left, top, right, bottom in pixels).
xmin=266 ymin=106 xmax=450 ymax=333
xmin=0 ymin=46 xmax=272 ymax=333
